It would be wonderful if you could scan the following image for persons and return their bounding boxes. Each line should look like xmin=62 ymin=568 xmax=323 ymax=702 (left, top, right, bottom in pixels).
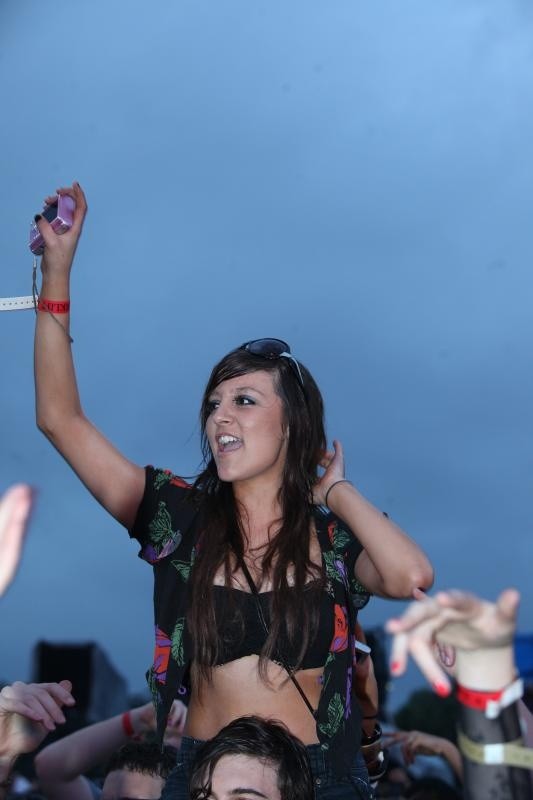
xmin=0 ymin=484 xmax=533 ymax=800
xmin=33 ymin=180 xmax=434 ymax=800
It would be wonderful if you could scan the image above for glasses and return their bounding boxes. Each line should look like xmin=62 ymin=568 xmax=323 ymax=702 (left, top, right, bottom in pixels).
xmin=241 ymin=338 xmax=304 ymax=388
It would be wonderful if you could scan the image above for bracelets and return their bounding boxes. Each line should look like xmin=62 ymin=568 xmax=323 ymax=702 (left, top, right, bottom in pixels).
xmin=122 ymin=711 xmax=132 ymax=736
xmin=458 ymin=728 xmax=533 ymax=770
xmin=325 ymin=480 xmax=351 ymax=508
xmin=458 ymin=673 xmax=524 ymax=719
xmin=0 ymin=296 xmax=70 ymax=314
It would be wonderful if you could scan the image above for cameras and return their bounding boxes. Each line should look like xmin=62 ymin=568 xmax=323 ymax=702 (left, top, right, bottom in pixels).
xmin=28 ymin=193 xmax=77 ymax=255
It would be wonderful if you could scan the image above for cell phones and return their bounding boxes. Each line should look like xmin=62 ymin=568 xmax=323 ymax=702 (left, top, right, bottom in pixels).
xmin=355 ymin=640 xmax=373 ymax=666
